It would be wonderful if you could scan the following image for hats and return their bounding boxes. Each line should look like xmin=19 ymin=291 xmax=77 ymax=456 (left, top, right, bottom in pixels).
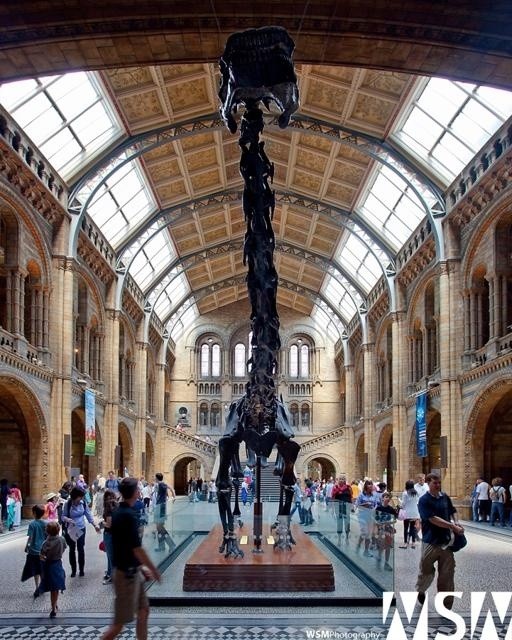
xmin=46 ymin=492 xmax=57 ymax=502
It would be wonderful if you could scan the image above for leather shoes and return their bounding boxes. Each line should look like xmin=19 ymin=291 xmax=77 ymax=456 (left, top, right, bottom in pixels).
xmin=418 ymin=592 xmax=426 ymax=604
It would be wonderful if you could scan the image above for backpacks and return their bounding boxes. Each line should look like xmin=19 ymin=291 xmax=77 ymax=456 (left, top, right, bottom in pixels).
xmin=490 ymin=486 xmax=502 ymax=500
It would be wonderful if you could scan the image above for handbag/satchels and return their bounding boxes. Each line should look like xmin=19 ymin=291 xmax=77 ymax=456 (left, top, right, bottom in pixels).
xmin=440 ymin=523 xmax=467 ymax=552
xmin=398 ymin=501 xmax=407 ymax=520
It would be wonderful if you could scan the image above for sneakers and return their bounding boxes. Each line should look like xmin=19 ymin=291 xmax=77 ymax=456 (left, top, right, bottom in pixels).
xmin=103 ymin=577 xmax=112 ymax=584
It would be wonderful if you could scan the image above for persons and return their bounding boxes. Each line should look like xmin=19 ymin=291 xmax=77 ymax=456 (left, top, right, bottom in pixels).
xmin=0 ymin=468 xmax=178 ymax=640
xmin=290 ymin=471 xmax=430 ymax=572
xmin=176 ymin=423 xmax=182 ymax=431
xmin=416 ymin=472 xmax=465 ymax=622
xmin=470 ymin=477 xmax=512 ymax=528
xmin=188 ymin=462 xmax=256 ymax=507
